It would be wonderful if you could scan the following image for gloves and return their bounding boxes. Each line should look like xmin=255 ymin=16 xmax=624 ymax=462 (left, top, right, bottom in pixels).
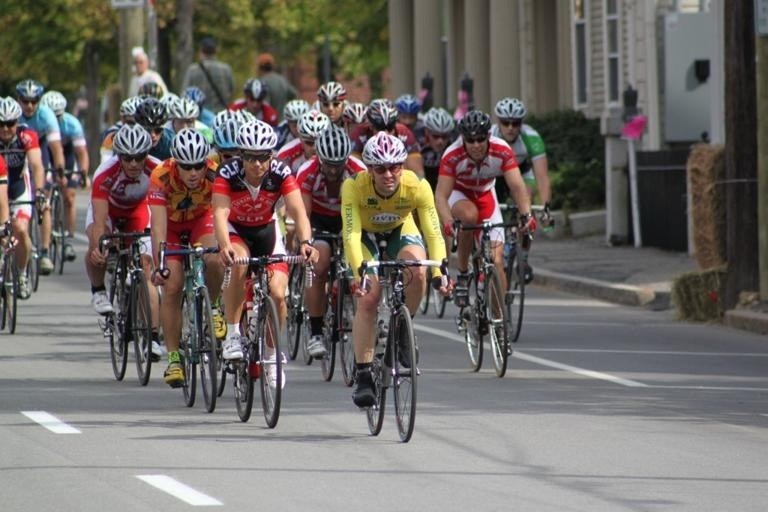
xmin=431 ymin=274 xmax=457 ymax=296
xmin=520 ymin=213 xmax=538 ymax=234
xmin=348 ymin=275 xmax=373 ymax=295
xmin=443 ymin=218 xmax=455 ymax=240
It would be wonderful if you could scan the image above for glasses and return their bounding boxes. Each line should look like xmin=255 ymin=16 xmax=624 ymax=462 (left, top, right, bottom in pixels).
xmin=304 ymin=141 xmax=315 ymax=147
xmin=463 ymin=133 xmax=486 ymax=144
xmin=371 ymin=166 xmax=403 ymax=175
xmin=500 ymin=121 xmax=522 ymax=128
xmin=220 ymin=150 xmax=241 ymax=160
xmin=145 ymin=128 xmax=165 ymax=135
xmin=321 ymin=101 xmax=341 ymax=108
xmin=0 ymin=119 xmax=18 ymax=128
xmin=241 ymin=153 xmax=271 ymax=162
xmin=176 ymin=161 xmax=206 ymax=170
xmin=18 ymin=98 xmax=37 ymax=105
xmin=432 ymin=135 xmax=448 ymax=140
xmin=118 ymin=153 xmax=147 ymax=162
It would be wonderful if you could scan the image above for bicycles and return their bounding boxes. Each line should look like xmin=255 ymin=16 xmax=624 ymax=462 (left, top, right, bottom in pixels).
xmin=354 ymin=258 xmax=449 ymax=443
xmin=451 ymin=213 xmax=534 ymax=379
xmin=0 ymin=167 xmax=87 ymax=335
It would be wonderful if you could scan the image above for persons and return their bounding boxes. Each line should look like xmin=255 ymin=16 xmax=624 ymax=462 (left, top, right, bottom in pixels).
xmin=84 ymin=82 xmax=555 ymax=409
xmin=131 ymin=38 xmax=298 ymax=124
xmin=1 ymin=78 xmax=89 ymax=300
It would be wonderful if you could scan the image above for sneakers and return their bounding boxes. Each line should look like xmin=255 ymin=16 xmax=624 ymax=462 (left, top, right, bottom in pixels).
xmin=220 ymin=332 xmax=243 ymax=359
xmin=205 ymin=304 xmax=227 ymax=339
xmin=454 ymin=273 xmax=472 ymax=307
xmin=164 ymin=360 xmax=185 ymax=388
xmin=515 ymin=263 xmax=532 ymax=284
xmin=17 ymin=271 xmax=31 ymax=299
xmin=90 ymin=290 xmax=114 ymax=314
xmin=38 ymin=256 xmax=54 ymax=275
xmin=139 ymin=336 xmax=162 ymax=363
xmin=64 ymin=244 xmax=76 ymax=260
xmin=389 ymin=313 xmax=419 ymax=368
xmin=263 ymin=353 xmax=286 ymax=390
xmin=306 ymin=332 xmax=328 ymax=357
xmin=495 ymin=335 xmax=512 ymax=356
xmin=352 ymin=365 xmax=380 ymax=407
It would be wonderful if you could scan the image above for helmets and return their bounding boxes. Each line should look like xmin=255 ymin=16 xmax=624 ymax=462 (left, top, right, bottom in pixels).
xmin=230 ymin=108 xmax=257 ymax=124
xmin=457 ymin=110 xmax=491 ymax=138
xmin=158 ymin=93 xmax=180 ymax=120
xmin=423 ymin=106 xmax=456 ymax=136
xmin=314 ymin=129 xmax=351 ymax=165
xmin=169 ymin=128 xmax=209 ymax=165
xmin=212 ymin=108 xmax=236 ymax=132
xmin=119 ymin=96 xmax=144 ymax=126
xmin=134 ymin=99 xmax=168 ymax=127
xmin=243 ymin=76 xmax=267 ymax=102
xmin=235 ymin=119 xmax=278 ymax=151
xmin=341 ymin=103 xmax=369 ymax=125
xmin=213 ymin=119 xmax=244 ymax=149
xmin=171 ymin=97 xmax=200 ymax=120
xmin=40 ymin=91 xmax=67 ymax=117
xmin=366 ymin=98 xmax=400 ymax=128
xmin=0 ymin=96 xmax=22 ymax=122
xmin=393 ymin=94 xmax=422 ymax=114
xmin=494 ymin=96 xmax=528 ymax=122
xmin=283 ymin=99 xmax=310 ymax=121
xmin=316 ymin=82 xmax=349 ymax=102
xmin=296 ymin=109 xmax=331 ymax=141
xmin=112 ymin=123 xmax=153 ymax=157
xmin=362 ymin=131 xmax=408 ymax=169
xmin=137 ymin=82 xmax=163 ymax=101
xmin=15 ymin=78 xmax=44 ymax=102
xmin=181 ymin=87 xmax=206 ymax=107
